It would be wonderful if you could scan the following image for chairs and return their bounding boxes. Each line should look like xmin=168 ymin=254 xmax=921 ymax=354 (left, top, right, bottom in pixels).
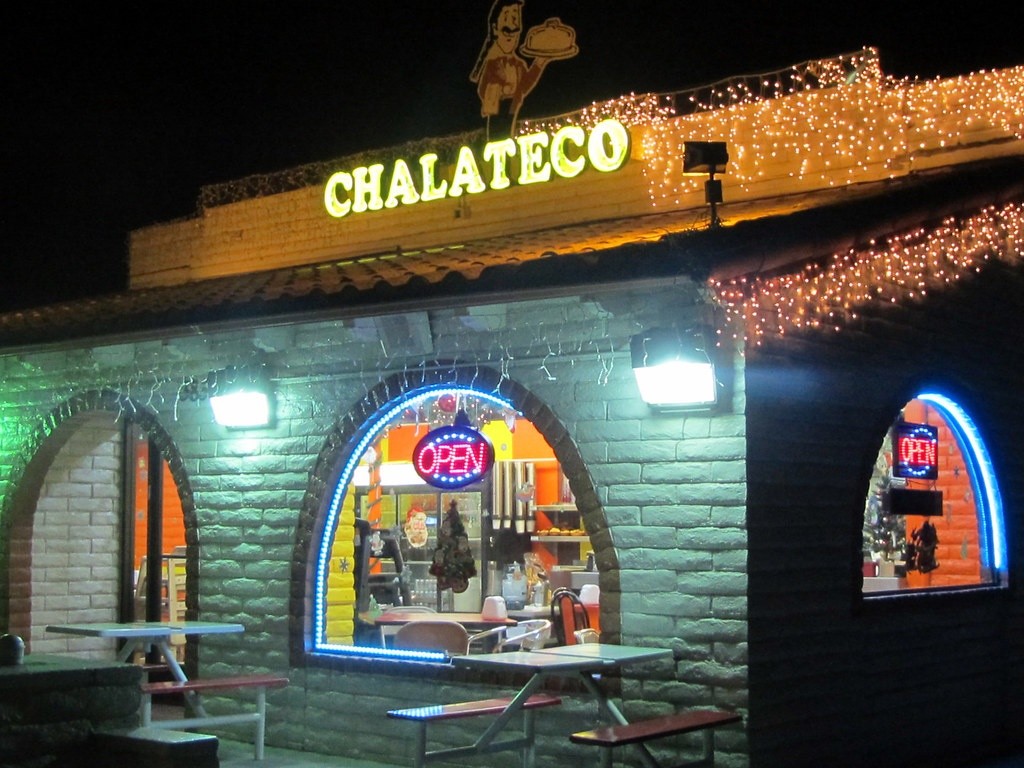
xmin=392 ymin=621 xmax=472 ymax=654
xmin=550 ymin=587 xmax=590 ymax=646
xmin=466 ymin=619 xmax=553 ymax=654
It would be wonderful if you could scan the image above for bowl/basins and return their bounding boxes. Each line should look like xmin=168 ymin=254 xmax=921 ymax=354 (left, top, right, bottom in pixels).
xmin=538 ymin=530 xmax=582 ymax=537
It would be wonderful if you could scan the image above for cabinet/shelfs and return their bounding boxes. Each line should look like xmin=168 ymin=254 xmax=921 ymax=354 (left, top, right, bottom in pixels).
xmin=531 ymin=505 xmax=589 ymax=542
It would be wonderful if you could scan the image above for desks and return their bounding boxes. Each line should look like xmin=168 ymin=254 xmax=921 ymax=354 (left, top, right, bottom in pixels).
xmin=373 ymin=611 xmax=517 ymax=651
xmin=45 ymin=621 xmax=266 ymax=763
xmin=417 ymin=641 xmax=716 ymax=768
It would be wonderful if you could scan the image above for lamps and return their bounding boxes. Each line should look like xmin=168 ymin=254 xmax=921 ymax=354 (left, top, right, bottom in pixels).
xmin=683 ymin=141 xmax=728 ymax=226
xmin=628 ymin=324 xmax=724 ymax=411
xmin=204 ymin=364 xmax=274 ymax=430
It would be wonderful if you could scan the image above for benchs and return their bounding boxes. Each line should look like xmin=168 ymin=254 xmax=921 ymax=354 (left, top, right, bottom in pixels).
xmin=137 ymin=675 xmax=290 ymax=689
xmin=568 ymin=710 xmax=742 ymax=748
xmin=385 ymin=694 xmax=562 ymax=720
